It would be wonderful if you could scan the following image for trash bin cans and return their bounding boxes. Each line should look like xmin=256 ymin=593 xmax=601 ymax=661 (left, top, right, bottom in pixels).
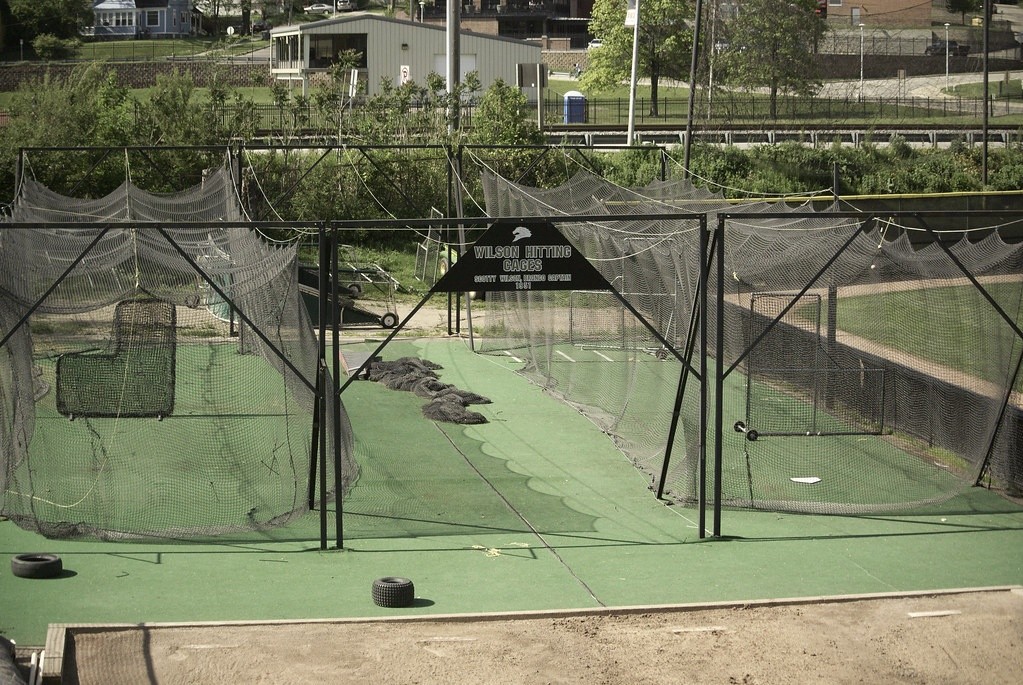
xmin=564 ymin=90 xmax=585 ymax=124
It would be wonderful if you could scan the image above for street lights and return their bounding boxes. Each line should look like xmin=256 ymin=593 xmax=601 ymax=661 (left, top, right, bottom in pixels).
xmin=945 ymin=23 xmax=951 ymax=92
xmin=859 ymin=23 xmax=866 ymax=100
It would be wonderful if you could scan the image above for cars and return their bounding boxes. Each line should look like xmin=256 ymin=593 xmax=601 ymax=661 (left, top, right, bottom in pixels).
xmin=587 ymin=39 xmax=604 ymax=50
xmin=253 ymin=22 xmax=267 ymax=34
xmin=981 ymin=4 xmax=998 ymax=14
xmin=304 ymin=4 xmax=334 ymax=15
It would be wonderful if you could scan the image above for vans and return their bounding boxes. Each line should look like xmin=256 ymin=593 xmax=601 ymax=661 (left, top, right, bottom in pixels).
xmin=338 ymin=0 xmax=358 ymax=11
